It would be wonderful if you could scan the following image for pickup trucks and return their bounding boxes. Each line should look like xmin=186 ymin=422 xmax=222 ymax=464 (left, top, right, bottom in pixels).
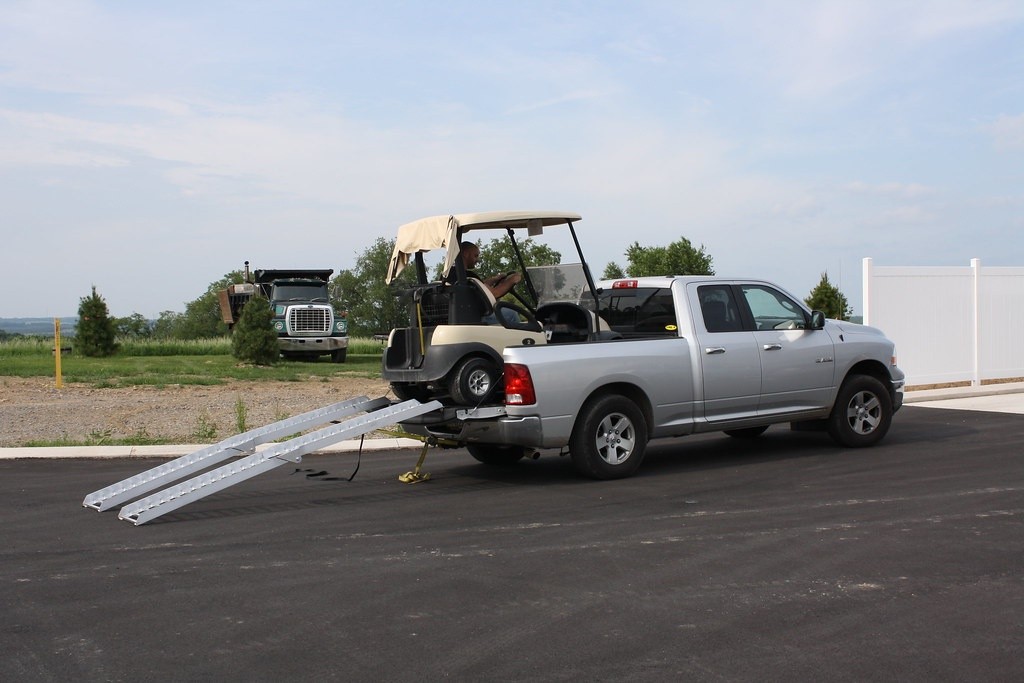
xmin=392 ymin=275 xmax=906 ymax=480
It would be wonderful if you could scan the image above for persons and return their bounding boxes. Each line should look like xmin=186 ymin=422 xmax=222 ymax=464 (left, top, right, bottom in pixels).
xmin=434 ymin=241 xmax=522 ymax=325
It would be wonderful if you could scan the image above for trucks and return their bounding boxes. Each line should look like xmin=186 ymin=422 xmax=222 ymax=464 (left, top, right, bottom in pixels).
xmin=223 ymin=268 xmax=349 ymax=362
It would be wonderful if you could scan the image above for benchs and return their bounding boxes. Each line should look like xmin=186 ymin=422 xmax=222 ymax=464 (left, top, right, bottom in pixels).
xmin=419 ymin=277 xmax=496 ymax=322
